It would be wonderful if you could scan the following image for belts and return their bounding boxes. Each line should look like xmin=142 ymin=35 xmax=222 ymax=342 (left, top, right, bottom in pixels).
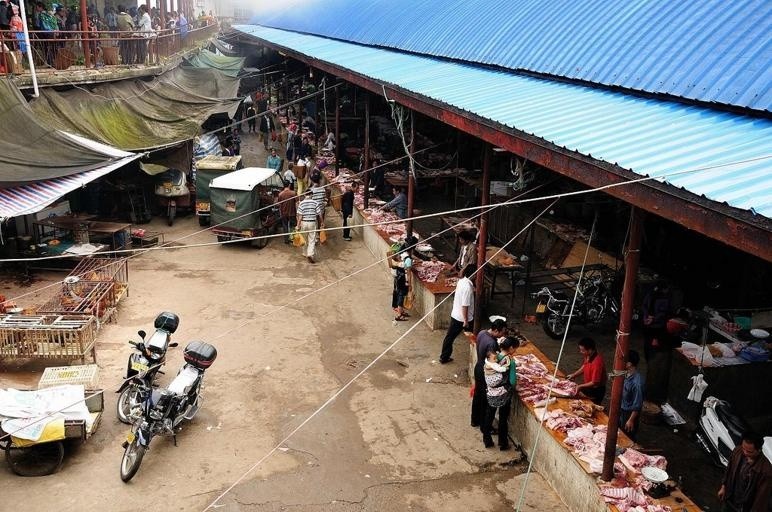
xmin=301 ymin=220 xmax=316 ymax=222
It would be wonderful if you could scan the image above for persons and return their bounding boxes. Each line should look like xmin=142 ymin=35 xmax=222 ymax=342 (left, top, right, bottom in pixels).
xmin=718 ymin=430 xmax=770 ymax=510
xmin=392 ymin=236 xmax=418 ymax=322
xmin=368 ymin=158 xmax=385 ymax=196
xmin=1 ymin=1 xmax=215 ymax=70
xmin=480 ymin=336 xmax=520 ymax=452
xmin=439 ymin=264 xmax=478 ymax=363
xmin=450 ymin=231 xmax=477 ymax=277
xmin=618 ymin=351 xmax=644 ymax=443
xmin=470 ymin=319 xmax=506 ymax=435
xmin=483 ymin=351 xmax=509 ymax=379
xmin=640 ymin=270 xmax=669 ymax=358
xmin=568 ymin=338 xmax=606 ymax=405
xmin=376 ymin=186 xmax=407 ymax=219
xmin=222 ymin=80 xmax=358 ymax=263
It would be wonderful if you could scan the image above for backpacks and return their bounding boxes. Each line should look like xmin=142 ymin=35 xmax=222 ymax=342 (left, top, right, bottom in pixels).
xmin=391 ymin=257 xmax=406 ymax=296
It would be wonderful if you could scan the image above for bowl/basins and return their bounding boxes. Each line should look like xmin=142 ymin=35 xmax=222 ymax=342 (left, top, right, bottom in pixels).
xmin=641 ymin=466 xmax=669 ymax=484
xmin=722 ymin=322 xmax=743 ymax=333
xmin=749 ymin=329 xmax=769 ymax=338
xmin=489 ymin=314 xmax=507 ymax=324
xmin=734 ymin=316 xmax=752 ymax=330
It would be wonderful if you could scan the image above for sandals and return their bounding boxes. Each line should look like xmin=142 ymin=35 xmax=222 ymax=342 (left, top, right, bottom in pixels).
xmin=395 ymin=310 xmax=411 ymax=321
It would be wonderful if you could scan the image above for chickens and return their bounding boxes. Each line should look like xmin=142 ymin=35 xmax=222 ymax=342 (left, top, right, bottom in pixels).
xmin=58 ymin=270 xmax=126 ymax=318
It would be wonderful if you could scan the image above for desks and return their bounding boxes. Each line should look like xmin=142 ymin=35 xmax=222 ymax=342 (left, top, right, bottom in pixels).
xmin=34 ymin=212 xmax=132 ymax=257
xmin=469 ymin=243 xmax=518 ymax=306
xmin=438 ymin=213 xmax=480 ymax=251
xmin=133 ymin=232 xmax=166 ymax=250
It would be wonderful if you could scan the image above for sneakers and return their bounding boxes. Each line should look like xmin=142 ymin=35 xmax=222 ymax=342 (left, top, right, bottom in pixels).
xmin=440 ymin=355 xmax=454 ymax=363
xmin=343 ymin=234 xmax=352 ymax=240
xmin=285 ymin=239 xmax=293 ymax=244
xmin=301 ymin=253 xmax=316 ymax=264
xmin=470 ymin=418 xmax=510 ymax=450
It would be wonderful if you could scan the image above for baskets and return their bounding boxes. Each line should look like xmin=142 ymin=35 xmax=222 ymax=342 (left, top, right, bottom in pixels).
xmin=293 ymin=165 xmax=307 ymax=179
xmin=386 ymin=250 xmax=399 ymax=269
xmin=54 ymin=48 xmax=77 ymax=70
xmin=5 ymin=50 xmax=23 ymax=75
xmin=330 ymin=194 xmax=343 ymax=212
xmin=100 ymin=44 xmax=120 ymax=65
xmin=737 ymin=343 xmax=769 ymax=363
xmin=37 ymin=363 xmax=100 ymax=392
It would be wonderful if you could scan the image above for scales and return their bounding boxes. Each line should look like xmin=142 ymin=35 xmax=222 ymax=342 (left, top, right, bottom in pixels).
xmin=740 ymin=329 xmax=770 ymax=362
xmin=374 ymin=200 xmax=386 ymax=209
xmin=489 ymin=316 xmax=506 ymax=326
xmin=642 ymin=467 xmax=671 ymax=499
xmin=415 ymin=244 xmax=437 ymax=260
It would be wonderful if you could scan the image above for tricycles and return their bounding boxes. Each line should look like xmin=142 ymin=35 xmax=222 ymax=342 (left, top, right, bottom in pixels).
xmin=2 ymin=388 xmax=103 ymax=477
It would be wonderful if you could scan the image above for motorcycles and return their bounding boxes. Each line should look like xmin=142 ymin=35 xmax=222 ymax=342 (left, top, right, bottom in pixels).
xmin=151 ymin=169 xmax=195 ymax=226
xmin=114 ymin=312 xmax=180 ymax=425
xmin=532 ymin=253 xmax=619 ymax=338
xmin=208 ymin=167 xmax=289 ymax=249
xmin=194 ymin=156 xmax=246 ymax=227
xmin=691 ymin=395 xmax=772 ymax=472
xmin=119 ymin=340 xmax=215 ymax=482
